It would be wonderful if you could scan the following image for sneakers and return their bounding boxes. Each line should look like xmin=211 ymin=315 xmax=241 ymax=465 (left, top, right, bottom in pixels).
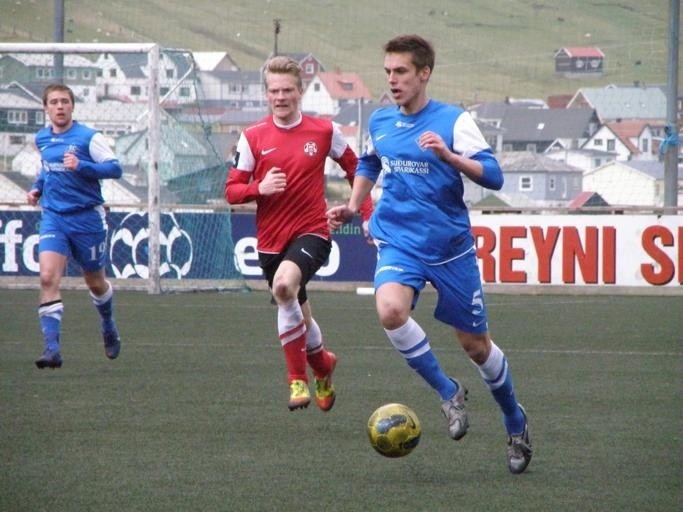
xmin=442 ymin=377 xmax=468 ymax=440
xmin=102 ymin=324 xmax=121 ymax=358
xmin=289 ymin=351 xmax=337 ymax=410
xmin=506 ymin=403 xmax=532 ymax=473
xmin=36 ymin=349 xmax=61 ymax=369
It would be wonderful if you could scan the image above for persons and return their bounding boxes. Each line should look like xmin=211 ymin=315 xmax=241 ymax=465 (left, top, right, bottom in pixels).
xmin=323 ymin=34 xmax=533 ymax=477
xmin=222 ymin=53 xmax=375 ymax=413
xmin=24 ymin=82 xmax=123 ymax=371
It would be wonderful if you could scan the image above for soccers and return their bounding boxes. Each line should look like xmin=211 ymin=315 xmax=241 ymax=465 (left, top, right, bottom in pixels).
xmin=367 ymin=403 xmax=421 ymax=457
